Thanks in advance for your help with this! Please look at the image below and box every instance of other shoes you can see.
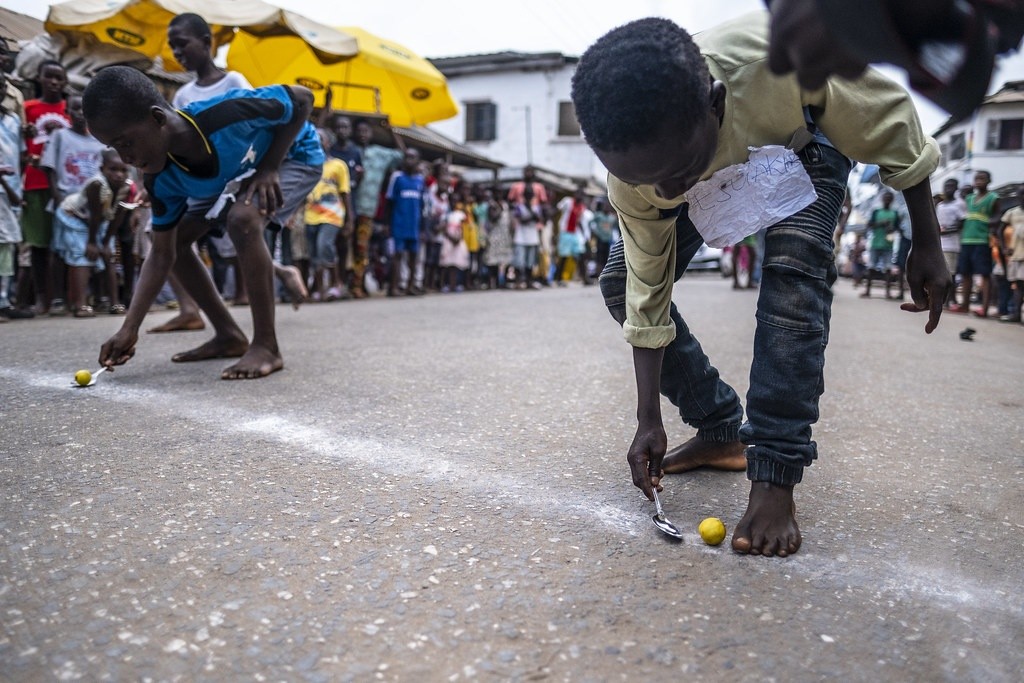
[948,304,969,313]
[971,306,987,318]
[1,305,34,319]
[109,303,126,314]
[73,305,96,316]
[998,313,1021,323]
[47,297,69,316]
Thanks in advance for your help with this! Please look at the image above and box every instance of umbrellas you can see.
[47,3,458,128]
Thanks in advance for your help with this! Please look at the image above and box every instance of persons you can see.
[858,191,900,301]
[57,150,135,317]
[897,201,912,299]
[933,194,940,206]
[146,94,618,311]
[935,179,965,310]
[768,1,1023,96]
[949,169,1002,317]
[143,13,306,334]
[998,190,1023,322]
[849,234,866,288]
[40,95,108,314]
[833,186,851,255]
[958,184,974,200]
[0,37,29,311]
[571,13,954,558]
[732,234,757,291]
[81,65,313,379]
[989,209,1013,316]
[0,72,39,321]
[21,59,73,316]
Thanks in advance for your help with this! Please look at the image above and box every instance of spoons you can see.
[71,365,110,386]
[649,483,684,541]
